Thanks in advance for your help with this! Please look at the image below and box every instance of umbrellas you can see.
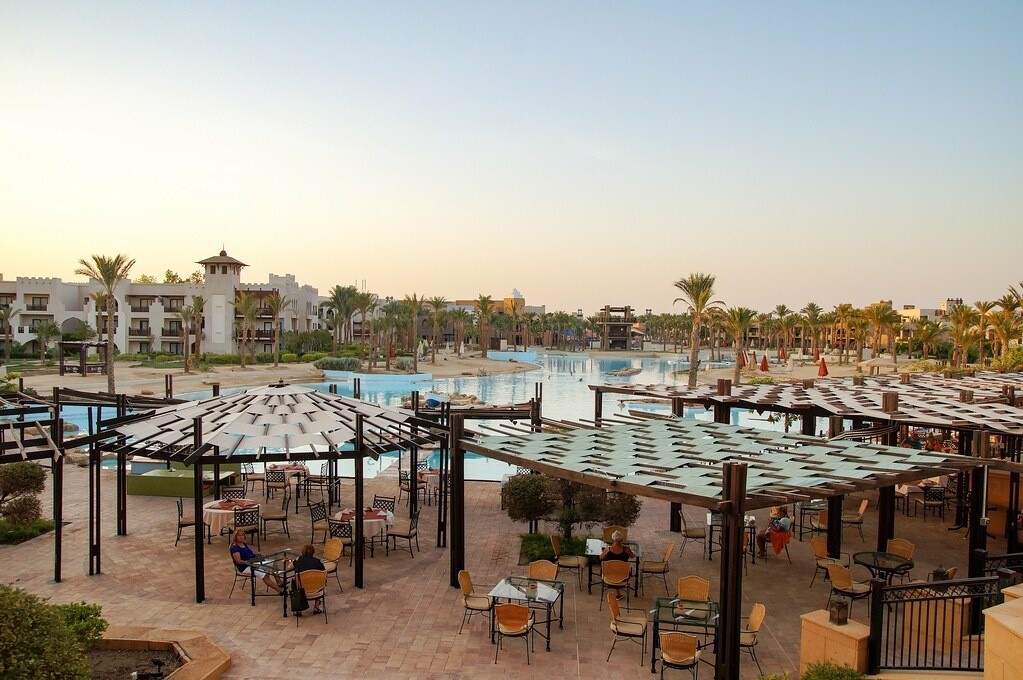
[387,342,396,357]
[759,355,770,374]
[798,348,803,363]
[858,357,900,375]
[817,357,828,379]
[778,348,786,360]
[459,341,464,357]
[446,343,450,351]
[741,350,758,368]
[814,349,819,363]
[417,338,436,360]
[468,339,473,347]
[833,341,845,347]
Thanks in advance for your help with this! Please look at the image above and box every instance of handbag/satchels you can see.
[289,573,309,612]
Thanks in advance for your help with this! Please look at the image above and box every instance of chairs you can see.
[229,538,344,628]
[367,494,396,546]
[550,535,588,591]
[458,570,496,638]
[221,485,245,535]
[387,506,422,558]
[308,499,337,546]
[526,560,558,617]
[397,459,450,507]
[265,468,292,503]
[327,517,366,567]
[307,461,329,497]
[494,604,535,666]
[288,460,306,490]
[599,429,1023,680]
[243,463,267,496]
[174,497,210,546]
[260,491,292,542]
[228,505,261,552]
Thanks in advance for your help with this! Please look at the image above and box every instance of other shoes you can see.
[278,586,289,596]
[279,581,290,588]
[756,554,765,559]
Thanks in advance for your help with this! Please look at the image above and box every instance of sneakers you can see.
[616,594,624,600]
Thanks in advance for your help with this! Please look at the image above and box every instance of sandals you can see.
[293,611,302,617]
[313,607,322,615]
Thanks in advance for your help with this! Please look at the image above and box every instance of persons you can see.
[600,530,636,601]
[923,432,943,455]
[230,529,291,596]
[288,543,328,617]
[756,505,792,558]
[899,434,922,453]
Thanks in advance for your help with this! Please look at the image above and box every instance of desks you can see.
[244,549,332,617]
[852,551,915,612]
[409,468,450,506]
[585,538,642,597]
[650,597,719,680]
[334,508,395,558]
[793,499,844,543]
[705,512,757,564]
[263,465,310,498]
[486,576,564,652]
[203,498,261,551]
[894,479,945,517]
